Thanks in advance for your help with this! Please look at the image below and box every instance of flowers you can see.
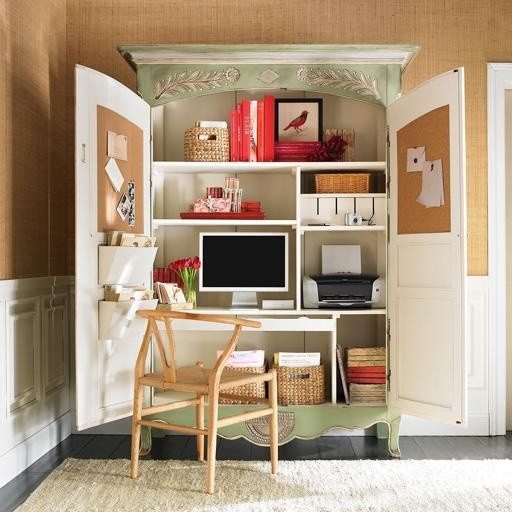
[167,256,200,291]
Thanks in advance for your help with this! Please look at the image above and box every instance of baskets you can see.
[314,173,369,193]
[273,364,324,406]
[184,128,229,162]
[218,360,267,404]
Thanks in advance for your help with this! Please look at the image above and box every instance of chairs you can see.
[129,308,279,494]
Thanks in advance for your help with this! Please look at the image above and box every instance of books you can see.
[243,100,249,161]
[106,232,157,249]
[104,287,155,302]
[230,109,237,162]
[278,351,322,368]
[256,98,264,162]
[215,349,265,368]
[195,120,227,140]
[154,282,186,305]
[346,347,387,407]
[236,102,243,161]
[249,99,257,164]
[263,95,275,162]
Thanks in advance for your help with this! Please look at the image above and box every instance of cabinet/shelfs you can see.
[74,42,469,458]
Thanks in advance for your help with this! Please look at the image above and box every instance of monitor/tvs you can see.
[198,232,289,310]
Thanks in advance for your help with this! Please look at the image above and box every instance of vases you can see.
[181,277,197,308]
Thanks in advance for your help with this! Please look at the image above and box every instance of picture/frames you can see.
[274,97,324,141]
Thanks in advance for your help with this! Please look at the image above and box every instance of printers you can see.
[301,273,385,308]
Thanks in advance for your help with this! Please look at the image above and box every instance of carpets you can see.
[13,457,511,512]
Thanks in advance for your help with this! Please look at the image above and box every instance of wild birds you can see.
[282,110,310,134]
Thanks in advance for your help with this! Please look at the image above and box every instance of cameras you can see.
[344,212,362,227]
